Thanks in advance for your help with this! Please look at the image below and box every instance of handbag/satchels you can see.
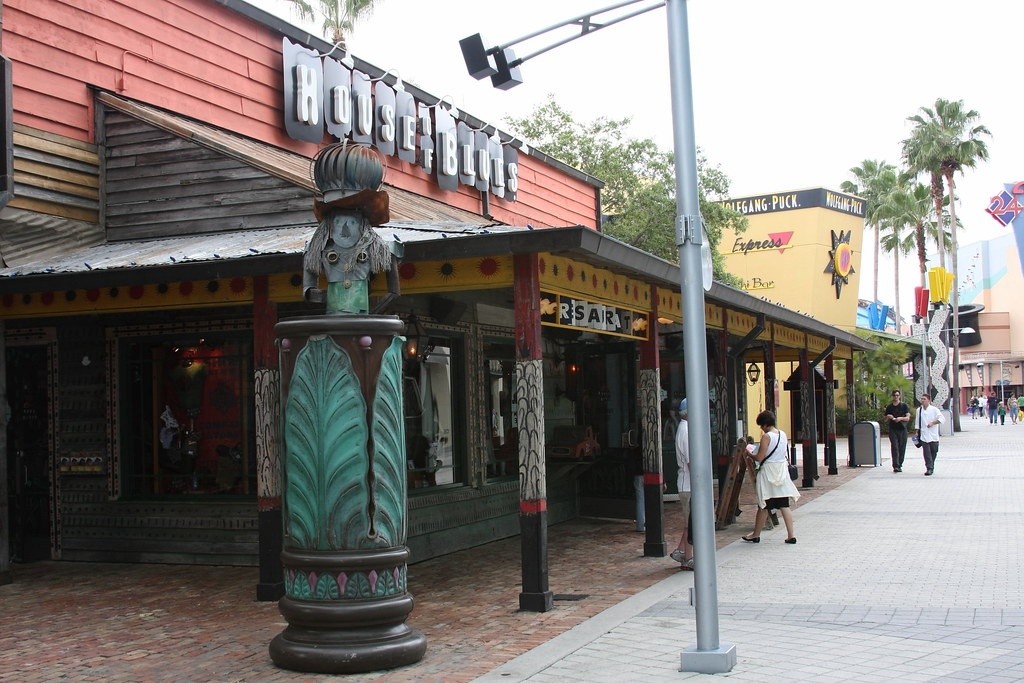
[1017,410,1023,421]
[787,464,798,480]
[915,435,922,448]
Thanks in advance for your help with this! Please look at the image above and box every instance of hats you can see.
[680,398,687,410]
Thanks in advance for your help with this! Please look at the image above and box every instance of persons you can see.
[670,396,700,571]
[884,389,911,472]
[969,392,1024,425]
[914,393,945,476]
[740,410,801,544]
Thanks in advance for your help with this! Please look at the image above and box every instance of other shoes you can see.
[898,467,902,472]
[1001,423,1004,425]
[925,469,933,475]
[894,468,899,472]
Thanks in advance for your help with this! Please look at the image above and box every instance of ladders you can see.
[716,437,779,530]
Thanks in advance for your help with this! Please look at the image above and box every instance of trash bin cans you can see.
[852,420,882,467]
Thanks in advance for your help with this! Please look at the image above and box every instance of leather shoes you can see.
[742,536,760,542]
[785,537,796,544]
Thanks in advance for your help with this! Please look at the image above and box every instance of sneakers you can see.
[681,558,695,570]
[670,549,686,562]
